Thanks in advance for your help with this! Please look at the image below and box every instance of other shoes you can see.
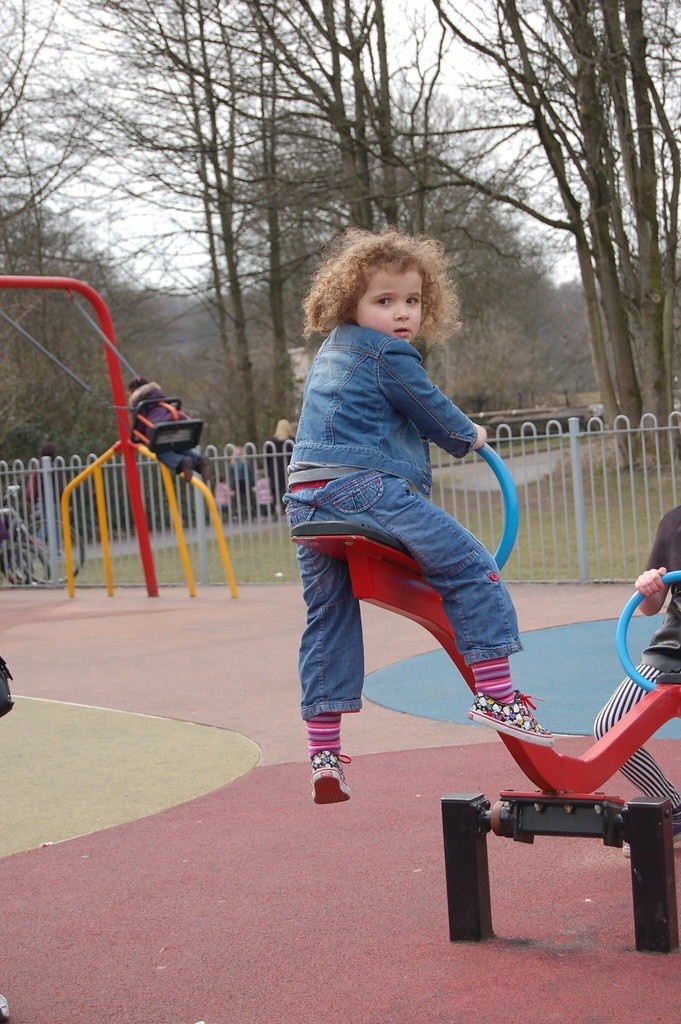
[255,515,264,523]
[266,516,274,524]
[622,805,681,858]
[180,456,193,482]
[197,456,211,481]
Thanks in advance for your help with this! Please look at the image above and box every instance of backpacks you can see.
[0,655,15,719]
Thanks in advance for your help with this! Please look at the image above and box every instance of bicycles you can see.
[0,484,85,586]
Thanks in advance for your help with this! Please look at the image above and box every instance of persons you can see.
[25,444,69,560]
[125,377,213,482]
[0,495,38,584]
[279,226,553,804]
[593,431,681,857]
[211,419,296,523]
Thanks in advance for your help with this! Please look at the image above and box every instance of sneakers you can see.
[310,750,352,804]
[467,689,554,747]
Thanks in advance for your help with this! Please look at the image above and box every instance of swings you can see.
[0,290,204,455]
